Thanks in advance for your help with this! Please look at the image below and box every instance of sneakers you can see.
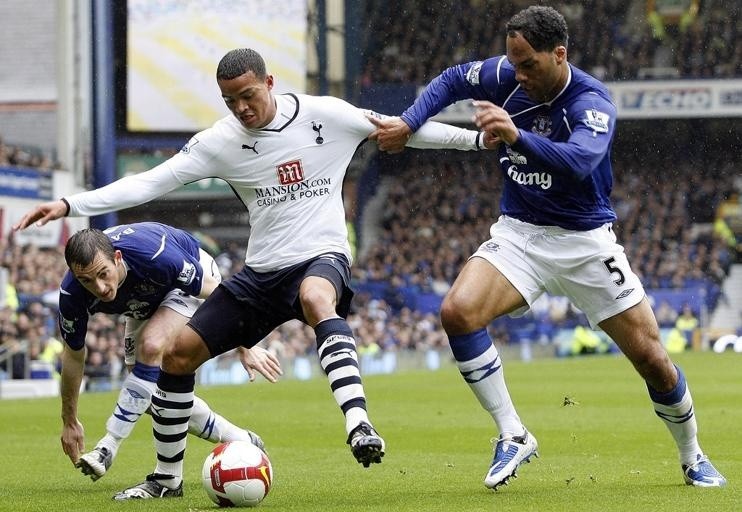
[112,473,183,500]
[248,431,265,454]
[77,447,111,482]
[347,422,385,468]
[485,425,539,491]
[682,454,727,487]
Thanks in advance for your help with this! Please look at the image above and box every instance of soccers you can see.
[203,441,273,506]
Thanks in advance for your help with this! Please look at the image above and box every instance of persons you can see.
[366,2,731,490]
[10,47,505,503]
[58,221,286,483]
